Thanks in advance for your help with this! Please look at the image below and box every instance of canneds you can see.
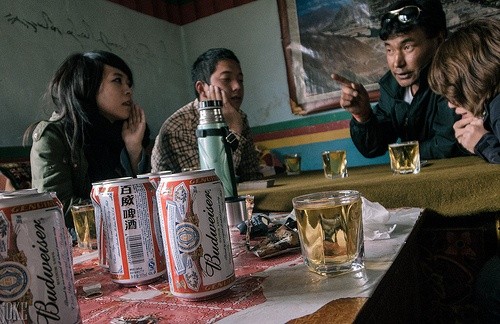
[90,168,235,302]
[0,187,80,324]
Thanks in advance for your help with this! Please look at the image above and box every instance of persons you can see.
[23,49,148,229]
[150,46,269,182]
[426,17,500,164]
[331,1,462,160]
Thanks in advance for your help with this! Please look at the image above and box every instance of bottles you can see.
[196,101,238,202]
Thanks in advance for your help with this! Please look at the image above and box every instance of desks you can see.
[0,156,500,324]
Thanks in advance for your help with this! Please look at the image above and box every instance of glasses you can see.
[381,6,426,25]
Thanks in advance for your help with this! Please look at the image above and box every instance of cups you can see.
[388,141,421,175]
[225,197,247,226]
[292,190,365,277]
[282,152,302,175]
[70,202,97,250]
[321,149,348,179]
